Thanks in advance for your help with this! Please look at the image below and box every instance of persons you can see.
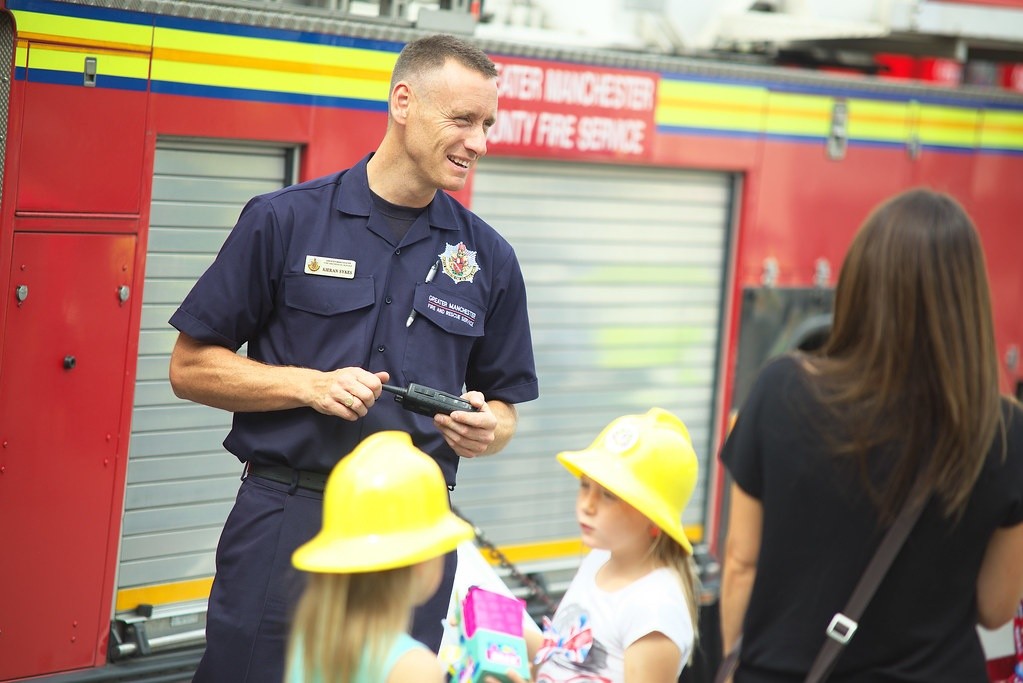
[281,431,474,683]
[481,406,703,683]
[717,188,1023,683]
[168,36,540,683]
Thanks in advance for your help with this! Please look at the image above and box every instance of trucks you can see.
[1,1,1023,683]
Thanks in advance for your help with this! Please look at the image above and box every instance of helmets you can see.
[557,407,698,556]
[291,428,475,575]
